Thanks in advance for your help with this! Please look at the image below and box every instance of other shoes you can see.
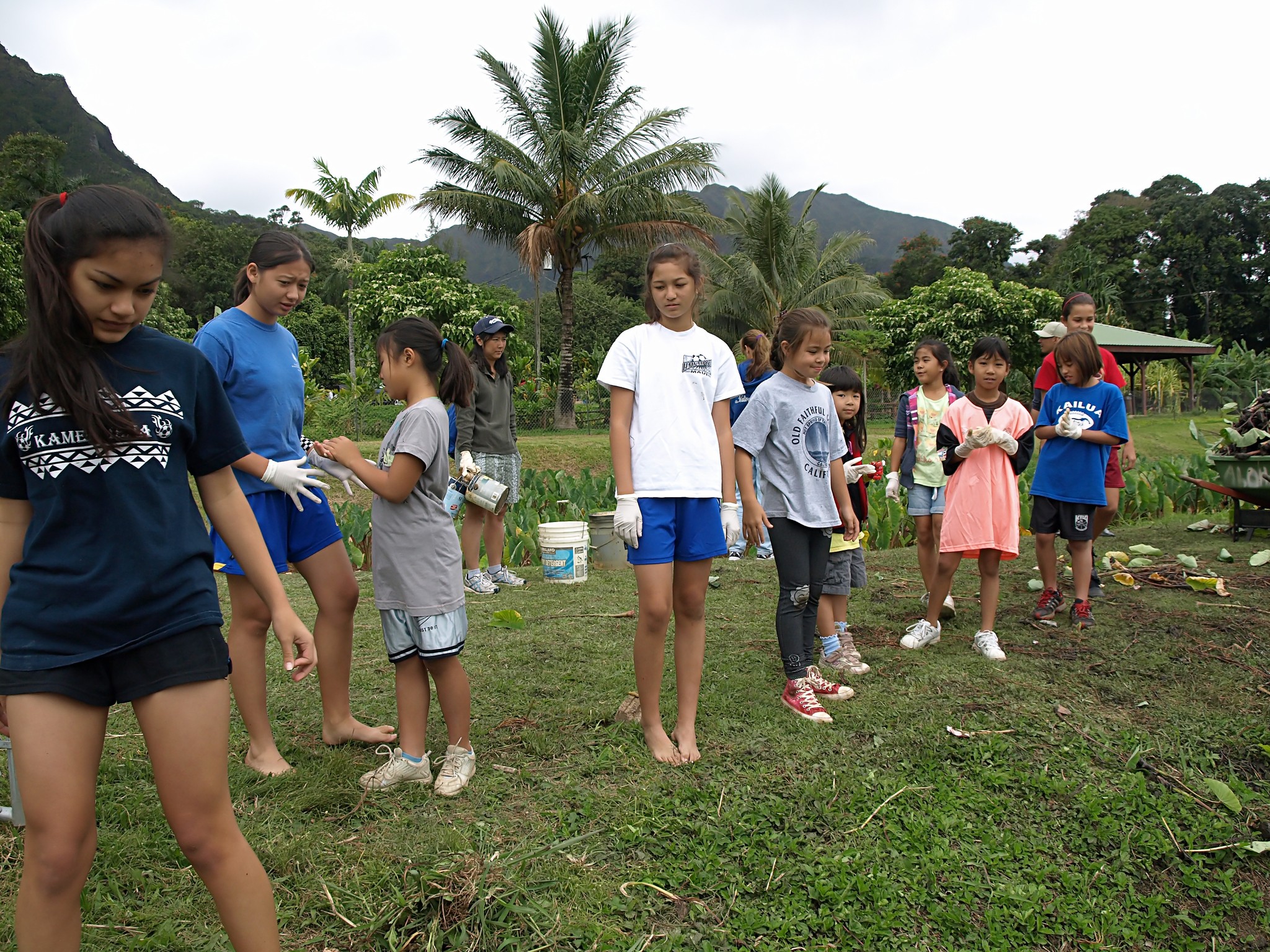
[1098,529,1115,537]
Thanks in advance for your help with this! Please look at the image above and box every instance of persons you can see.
[731,307,860,723]
[454,313,527,594]
[885,339,965,620]
[899,336,1034,661]
[1029,321,1115,537]
[729,329,779,560]
[312,317,477,797]
[596,242,740,767]
[818,365,883,675]
[1028,331,1129,628]
[0,183,319,952]
[1032,291,1136,598]
[191,231,397,777]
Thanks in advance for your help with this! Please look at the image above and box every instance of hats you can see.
[473,316,514,339]
[1032,322,1067,338]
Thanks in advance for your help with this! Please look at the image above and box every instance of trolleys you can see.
[1180,453,1270,540]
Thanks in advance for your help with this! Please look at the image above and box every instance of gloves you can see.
[867,461,883,480]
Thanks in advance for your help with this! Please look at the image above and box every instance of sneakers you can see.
[358,745,432,790]
[972,629,1007,661]
[899,619,941,649]
[1031,586,1068,621]
[1070,597,1096,629]
[1066,543,1097,569]
[1088,570,1105,597]
[805,665,854,700]
[818,647,871,675]
[489,566,526,586]
[728,551,744,560]
[756,554,774,560]
[433,736,476,796]
[837,632,861,660]
[463,570,501,595]
[780,677,834,724]
[920,590,957,618]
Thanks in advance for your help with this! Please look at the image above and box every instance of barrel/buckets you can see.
[443,475,469,519]
[465,472,510,515]
[588,511,632,571]
[537,499,590,584]
